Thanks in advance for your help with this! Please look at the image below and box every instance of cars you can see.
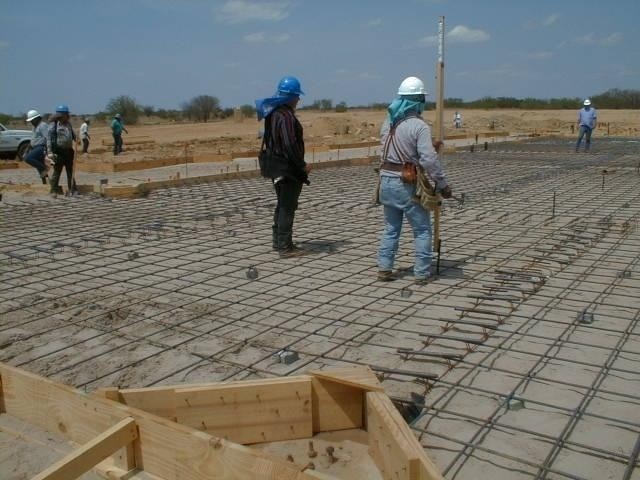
[0,121,36,162]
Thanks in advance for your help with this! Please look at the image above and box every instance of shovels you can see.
[65,127,80,198]
[57,185,64,195]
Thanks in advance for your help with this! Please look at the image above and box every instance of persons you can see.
[252,74,313,261]
[373,74,452,286]
[453,110,463,129]
[45,103,82,196]
[78,118,91,153]
[109,112,130,157]
[20,108,50,186]
[574,98,596,153]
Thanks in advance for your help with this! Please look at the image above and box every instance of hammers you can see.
[450,193,465,209]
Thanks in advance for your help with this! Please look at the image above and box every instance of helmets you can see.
[397,76,429,95]
[84,118,90,122]
[26,110,40,121]
[584,99,591,105]
[56,104,71,113]
[277,76,305,95]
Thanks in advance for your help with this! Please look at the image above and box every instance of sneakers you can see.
[377,270,393,281]
[40,165,50,178]
[415,277,428,286]
[50,190,82,199]
[272,244,305,259]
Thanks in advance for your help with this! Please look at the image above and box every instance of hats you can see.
[112,114,123,120]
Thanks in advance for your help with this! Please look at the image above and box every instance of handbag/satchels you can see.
[401,163,442,211]
[258,147,297,179]
[372,178,381,203]
